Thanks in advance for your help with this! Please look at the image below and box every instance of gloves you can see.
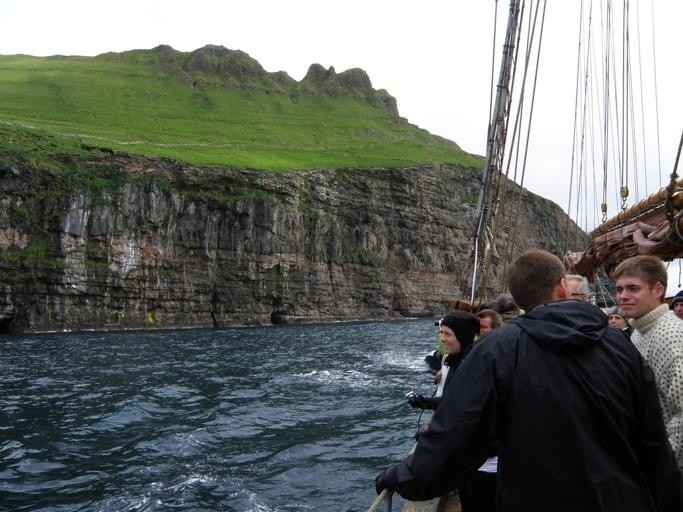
[372,464,397,499]
[407,394,424,410]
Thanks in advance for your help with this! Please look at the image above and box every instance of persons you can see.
[374,249,682,511]
[399,271,682,416]
[616,252,682,461]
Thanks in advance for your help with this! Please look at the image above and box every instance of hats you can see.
[606,305,628,325]
[440,309,480,346]
[671,289,683,309]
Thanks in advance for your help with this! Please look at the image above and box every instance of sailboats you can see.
[364,0,682,510]
